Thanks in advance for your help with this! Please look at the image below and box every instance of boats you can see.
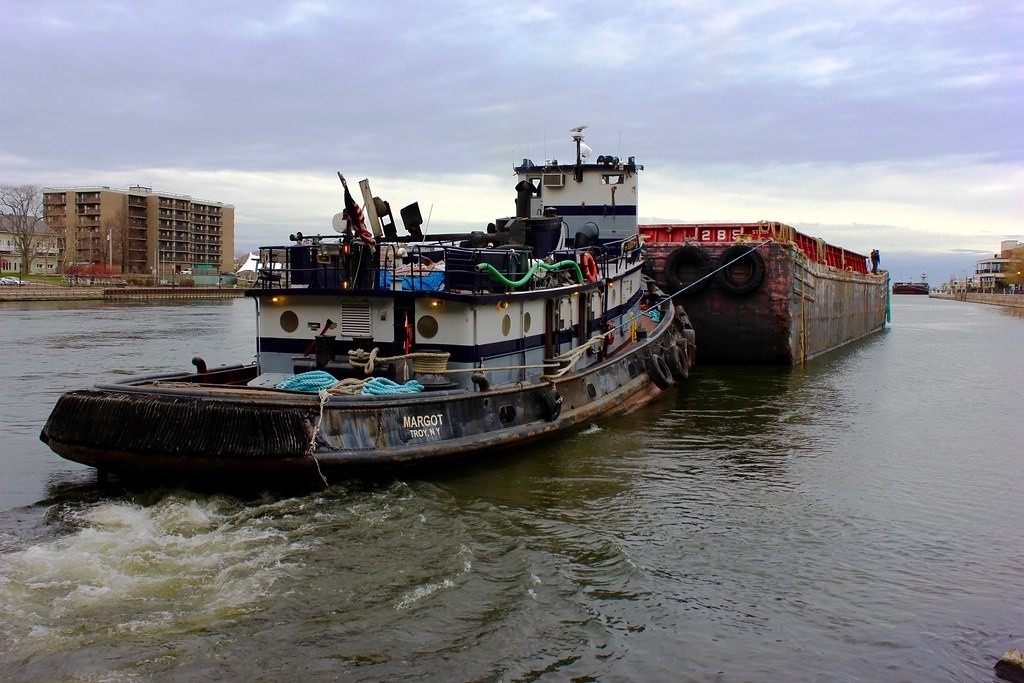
[39,128,697,480]
[892,282,929,295]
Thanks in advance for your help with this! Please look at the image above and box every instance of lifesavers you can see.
[582,252,599,284]
[605,322,616,344]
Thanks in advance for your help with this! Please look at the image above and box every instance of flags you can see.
[339,187,376,246]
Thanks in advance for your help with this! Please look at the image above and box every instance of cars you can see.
[1,276,26,286]
[181,268,192,275]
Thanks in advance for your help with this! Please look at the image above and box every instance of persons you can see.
[871,249,875,273]
[873,250,880,275]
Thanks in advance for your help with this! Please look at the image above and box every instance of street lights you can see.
[1018,272,1020,290]
